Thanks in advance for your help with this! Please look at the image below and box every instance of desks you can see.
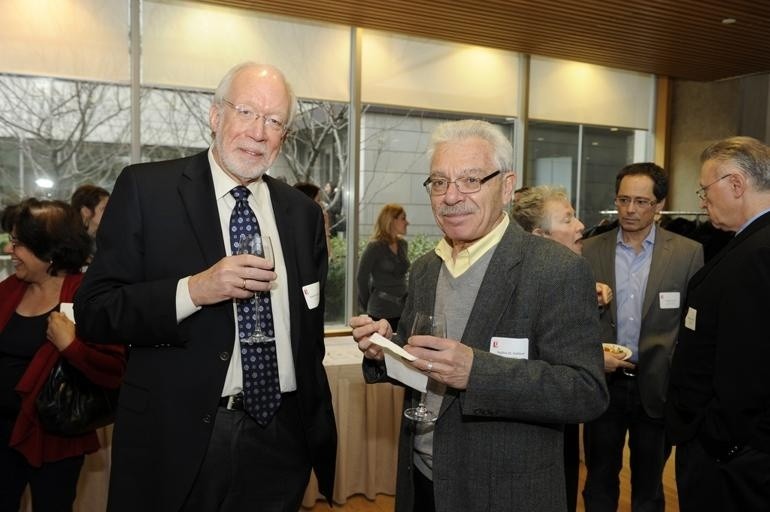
[304,335,406,506]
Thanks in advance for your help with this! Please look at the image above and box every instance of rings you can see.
[427,359,433,373]
[243,278,247,288]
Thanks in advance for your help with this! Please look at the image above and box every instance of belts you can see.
[218,390,297,412]
[616,363,639,377]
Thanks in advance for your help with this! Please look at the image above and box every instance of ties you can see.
[229,185,282,429]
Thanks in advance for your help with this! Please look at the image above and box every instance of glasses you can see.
[695,172,733,201]
[612,194,657,208]
[222,97,285,131]
[8,233,25,249]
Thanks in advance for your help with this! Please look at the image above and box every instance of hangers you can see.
[590,208,718,237]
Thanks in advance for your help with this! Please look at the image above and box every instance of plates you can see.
[603,341,632,360]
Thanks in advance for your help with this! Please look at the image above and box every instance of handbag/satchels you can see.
[33,359,120,438]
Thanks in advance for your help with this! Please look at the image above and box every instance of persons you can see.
[355,206,412,333]
[582,164,704,511]
[70,185,111,272]
[0,199,126,512]
[290,182,331,260]
[511,183,635,373]
[672,135,770,512]
[349,118,609,512]
[72,62,338,512]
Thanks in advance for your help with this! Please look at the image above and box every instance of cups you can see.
[405,313,447,424]
[239,235,276,344]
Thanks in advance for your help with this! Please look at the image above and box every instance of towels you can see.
[423,169,501,196]
